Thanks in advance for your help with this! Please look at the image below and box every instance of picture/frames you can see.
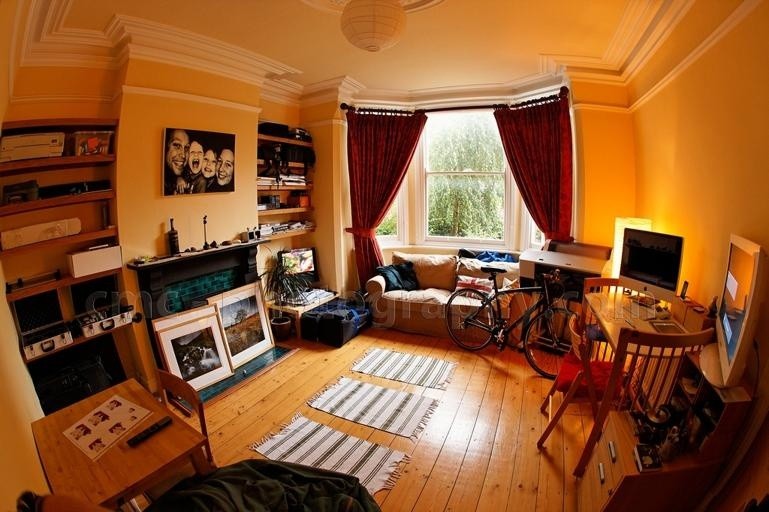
[157,313,235,393]
[151,304,220,333]
[207,281,275,368]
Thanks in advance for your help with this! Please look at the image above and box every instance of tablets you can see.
[650,321,684,335]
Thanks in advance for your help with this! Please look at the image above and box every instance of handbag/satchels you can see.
[300,296,373,348]
[375,261,420,293]
[258,139,316,181]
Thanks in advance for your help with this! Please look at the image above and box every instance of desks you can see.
[573,278,717,479]
[32,379,208,509]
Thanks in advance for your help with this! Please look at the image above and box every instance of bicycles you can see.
[444,266,579,379]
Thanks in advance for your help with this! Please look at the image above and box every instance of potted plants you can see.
[265,264,310,340]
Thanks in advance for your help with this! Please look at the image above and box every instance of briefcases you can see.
[12,288,74,361]
[69,274,135,339]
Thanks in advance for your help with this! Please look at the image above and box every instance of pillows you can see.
[456,276,495,299]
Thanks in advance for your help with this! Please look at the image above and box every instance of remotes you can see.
[126,416,173,446]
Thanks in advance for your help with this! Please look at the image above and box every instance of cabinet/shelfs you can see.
[0,115,135,377]
[519,249,604,353]
[260,121,317,268]
[577,353,752,512]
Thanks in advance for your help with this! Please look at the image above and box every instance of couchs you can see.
[364,251,522,341]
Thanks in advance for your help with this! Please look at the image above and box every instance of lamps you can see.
[340,0,407,52]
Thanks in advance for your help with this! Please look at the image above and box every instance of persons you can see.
[165,129,235,193]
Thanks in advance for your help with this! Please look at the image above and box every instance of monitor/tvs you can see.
[699,233,767,387]
[617,226,684,300]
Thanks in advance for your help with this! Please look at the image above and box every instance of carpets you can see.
[308,376,441,439]
[351,347,459,391]
[247,413,409,497]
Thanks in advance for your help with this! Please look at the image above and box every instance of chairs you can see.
[537,313,636,448]
[130,367,217,512]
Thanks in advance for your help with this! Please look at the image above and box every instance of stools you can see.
[268,290,338,340]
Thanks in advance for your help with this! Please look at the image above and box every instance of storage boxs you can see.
[70,131,112,156]
[63,246,123,279]
